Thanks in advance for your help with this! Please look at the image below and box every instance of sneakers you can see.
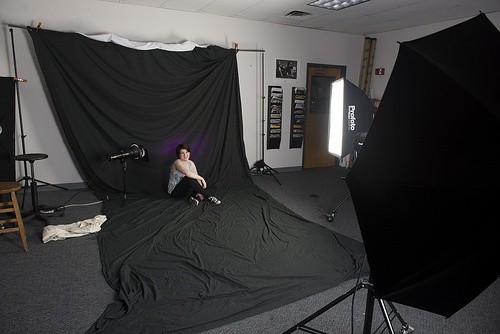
[189,197,199,206]
[208,196,221,205]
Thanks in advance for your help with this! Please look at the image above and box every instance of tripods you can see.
[9,28,68,209]
[249,53,282,186]
[105,159,140,206]
[282,271,415,334]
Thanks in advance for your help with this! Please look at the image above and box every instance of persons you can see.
[168,143,221,206]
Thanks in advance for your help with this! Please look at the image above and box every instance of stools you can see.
[0,182,31,253]
[14,153,65,227]
